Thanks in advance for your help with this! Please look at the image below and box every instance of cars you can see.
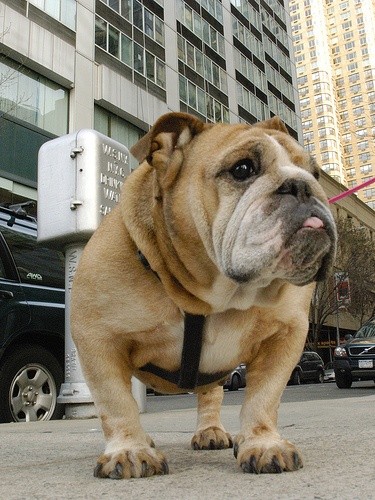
[225,364,249,391]
[284,352,325,386]
[324,369,336,381]
[331,322,375,388]
[0,206,65,423]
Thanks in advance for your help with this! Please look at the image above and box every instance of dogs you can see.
[68,111,340,481]
